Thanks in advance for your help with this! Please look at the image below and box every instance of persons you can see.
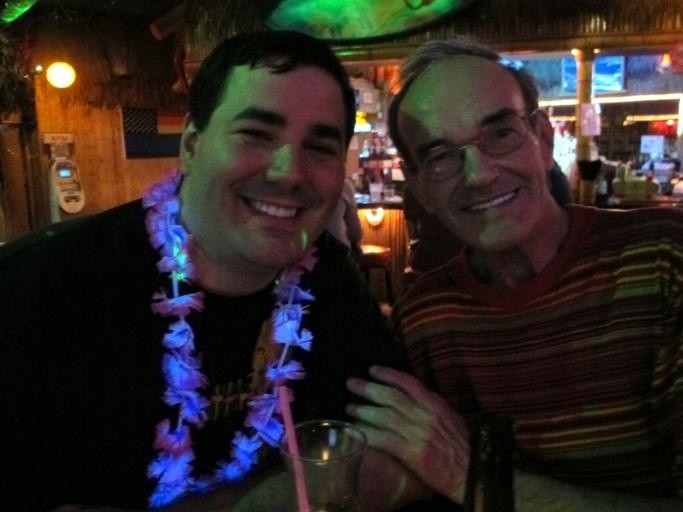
[341,35,683,512]
[397,183,460,303]
[546,156,573,211]
[1,29,439,512]
[325,179,370,284]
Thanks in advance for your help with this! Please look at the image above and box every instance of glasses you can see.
[403,106,540,184]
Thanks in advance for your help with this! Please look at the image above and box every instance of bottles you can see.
[359,138,392,166]
[461,413,517,512]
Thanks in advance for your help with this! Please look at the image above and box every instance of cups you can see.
[278,421,367,511]
[369,183,395,202]
[579,179,596,205]
